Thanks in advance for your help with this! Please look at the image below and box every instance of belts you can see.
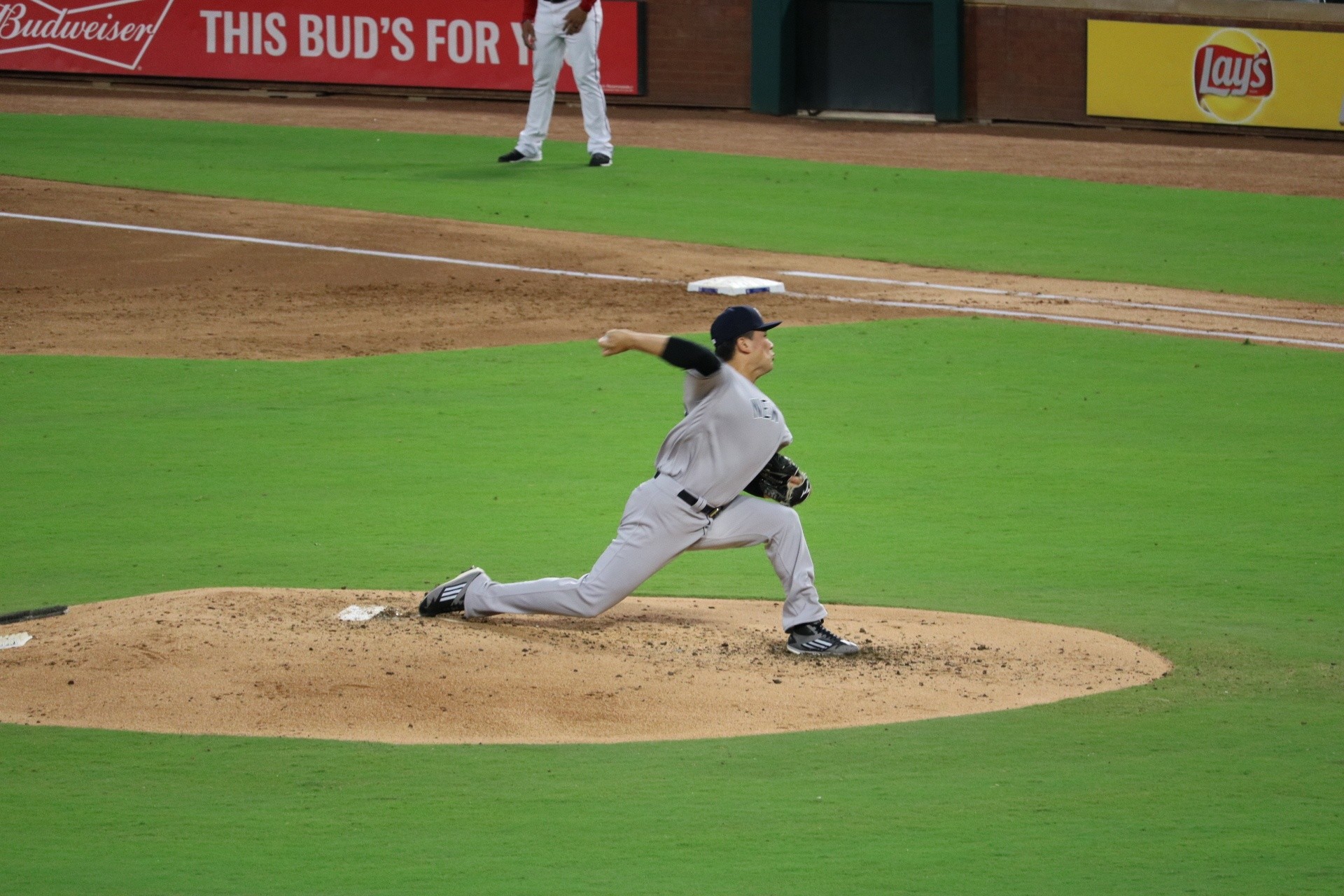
[655,470,724,520]
[544,0,568,3]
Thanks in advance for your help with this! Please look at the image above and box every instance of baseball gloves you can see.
[751,451,811,508]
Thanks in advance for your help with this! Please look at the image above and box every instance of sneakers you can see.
[786,618,859,656]
[419,567,486,616]
[498,149,542,163]
[588,153,612,167]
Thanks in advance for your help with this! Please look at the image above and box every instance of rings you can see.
[575,25,578,28]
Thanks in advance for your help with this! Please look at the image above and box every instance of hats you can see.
[711,306,782,347]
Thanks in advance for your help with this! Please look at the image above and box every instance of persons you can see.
[419,306,858,655]
[498,0,613,166]
